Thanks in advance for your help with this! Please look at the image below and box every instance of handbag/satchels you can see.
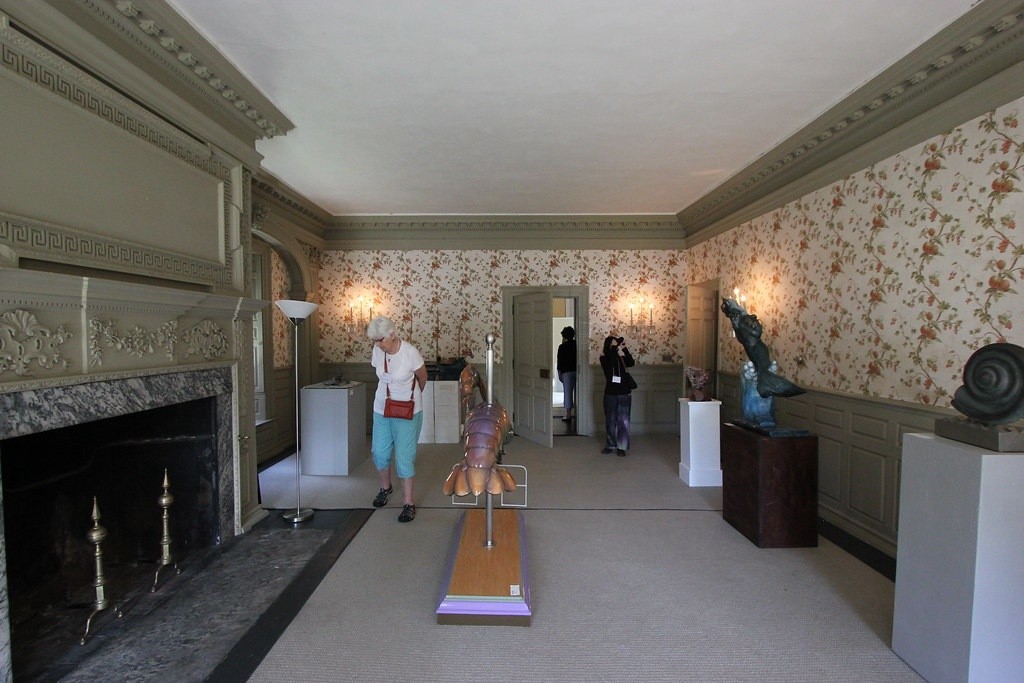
[383,397,415,421]
[620,356,638,389]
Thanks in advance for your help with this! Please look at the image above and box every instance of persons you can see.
[599,335,637,457]
[367,316,429,522]
[557,326,576,422]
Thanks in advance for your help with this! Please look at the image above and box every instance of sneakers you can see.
[373,483,393,507]
[398,502,416,522]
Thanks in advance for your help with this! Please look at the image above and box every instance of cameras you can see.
[614,337,624,346]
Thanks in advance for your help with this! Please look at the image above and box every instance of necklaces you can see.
[387,337,399,363]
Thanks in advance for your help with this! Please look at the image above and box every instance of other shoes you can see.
[600,447,614,454]
[617,449,626,457]
[561,415,571,421]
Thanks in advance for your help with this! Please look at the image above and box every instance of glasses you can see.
[372,336,385,343]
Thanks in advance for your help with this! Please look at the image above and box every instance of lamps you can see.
[273,299,319,524]
[346,297,374,333]
[627,296,656,335]
[728,287,762,338]
